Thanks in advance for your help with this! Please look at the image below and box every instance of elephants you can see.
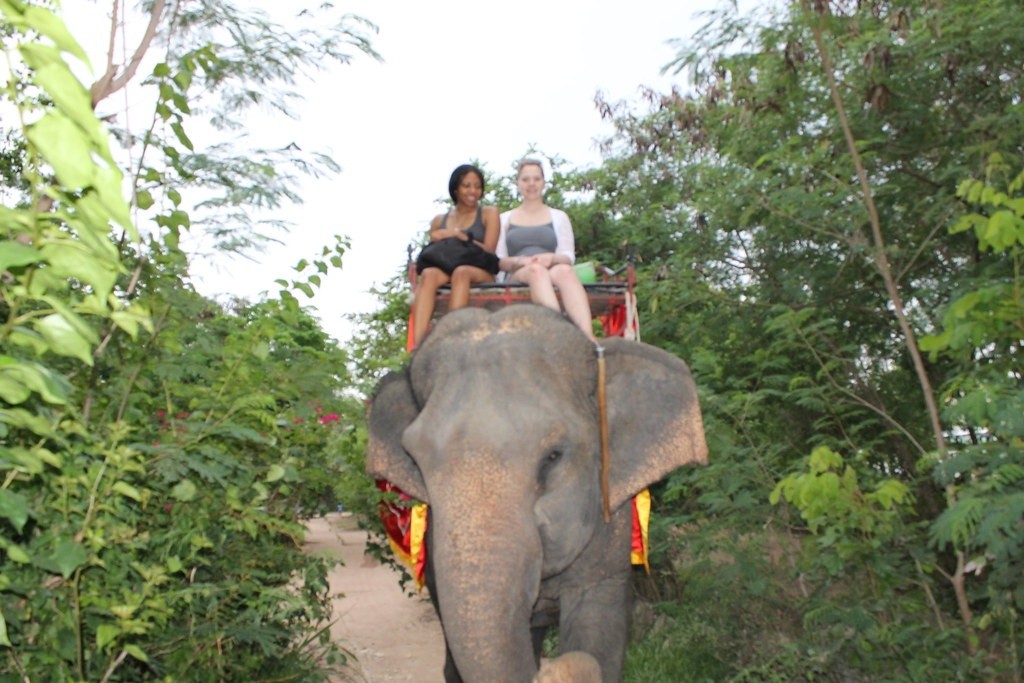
[362,300,711,683]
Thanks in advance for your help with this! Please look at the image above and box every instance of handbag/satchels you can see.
[415,232,499,274]
[572,261,596,282]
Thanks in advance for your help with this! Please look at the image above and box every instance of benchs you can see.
[407,260,640,350]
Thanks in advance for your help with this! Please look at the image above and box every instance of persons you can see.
[413,165,500,350]
[495,160,600,346]
